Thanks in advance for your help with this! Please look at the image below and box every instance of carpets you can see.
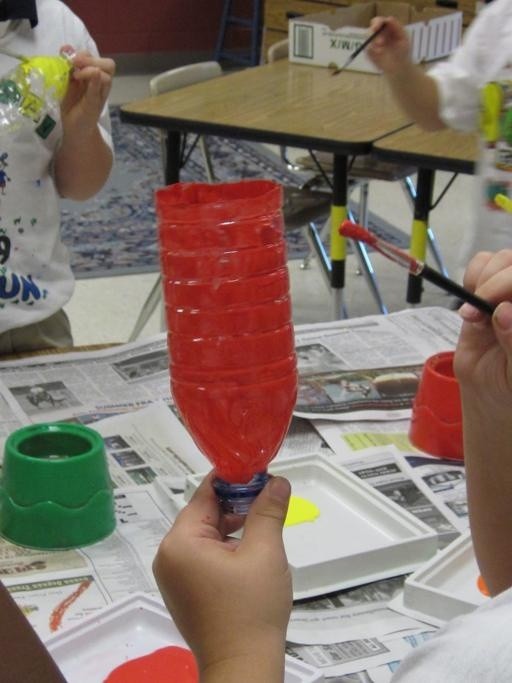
[56,101,412,280]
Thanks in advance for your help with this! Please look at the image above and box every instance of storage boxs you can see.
[286,1,464,74]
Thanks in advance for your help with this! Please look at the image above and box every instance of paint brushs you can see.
[338,219,495,317]
[332,21,388,76]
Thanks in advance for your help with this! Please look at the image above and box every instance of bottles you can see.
[407,351,464,461]
[154,179,299,515]
[0,51,78,143]
[0,422,117,551]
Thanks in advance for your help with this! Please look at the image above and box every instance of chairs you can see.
[125,57,386,347]
[265,35,454,294]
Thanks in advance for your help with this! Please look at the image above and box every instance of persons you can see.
[152,247,512,683]
[0,0,115,354]
[365,0,512,310]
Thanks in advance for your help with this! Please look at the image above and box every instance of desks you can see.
[2,337,512,681]
[116,55,414,320]
[372,118,496,312]
